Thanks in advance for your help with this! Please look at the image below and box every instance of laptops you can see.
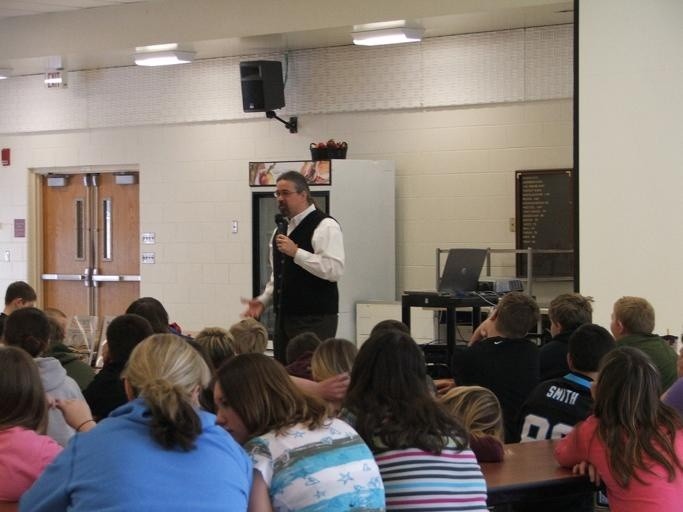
[403,248,488,296]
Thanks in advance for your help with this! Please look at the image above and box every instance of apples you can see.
[258,171,273,186]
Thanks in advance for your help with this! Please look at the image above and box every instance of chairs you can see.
[63,314,97,366]
[95,316,117,374]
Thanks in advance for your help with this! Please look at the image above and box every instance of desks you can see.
[403,295,498,378]
[478,440,575,494]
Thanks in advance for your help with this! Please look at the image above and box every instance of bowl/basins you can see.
[310,140,348,161]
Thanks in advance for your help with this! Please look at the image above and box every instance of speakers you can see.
[239,60,285,112]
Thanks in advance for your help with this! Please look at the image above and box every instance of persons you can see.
[0,282,682,510]
[240,172,345,370]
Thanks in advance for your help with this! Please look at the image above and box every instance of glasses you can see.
[273,189,303,198]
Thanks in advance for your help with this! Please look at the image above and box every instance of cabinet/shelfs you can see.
[356,302,548,352]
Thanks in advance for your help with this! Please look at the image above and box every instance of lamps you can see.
[351,28,425,47]
[135,51,197,67]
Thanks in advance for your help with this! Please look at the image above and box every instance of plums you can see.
[317,139,347,148]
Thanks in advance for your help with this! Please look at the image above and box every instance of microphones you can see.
[274,214,289,263]
